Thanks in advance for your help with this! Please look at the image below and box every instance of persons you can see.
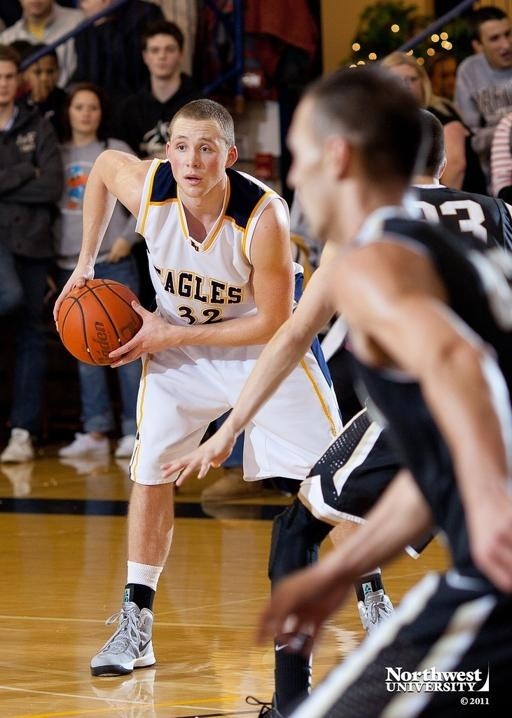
[46,82,154,461]
[156,106,512,716]
[24,41,59,106]
[49,97,395,678]
[376,51,491,197]
[111,20,202,159]
[1,45,66,463]
[246,67,512,716]
[0,1,85,93]
[453,5,512,133]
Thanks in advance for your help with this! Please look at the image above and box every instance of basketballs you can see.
[58,279,141,364]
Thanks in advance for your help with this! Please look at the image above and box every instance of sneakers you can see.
[58,432,109,457]
[90,601,156,677]
[115,435,136,458]
[357,595,395,633]
[0,428,35,462]
[245,693,311,718]
